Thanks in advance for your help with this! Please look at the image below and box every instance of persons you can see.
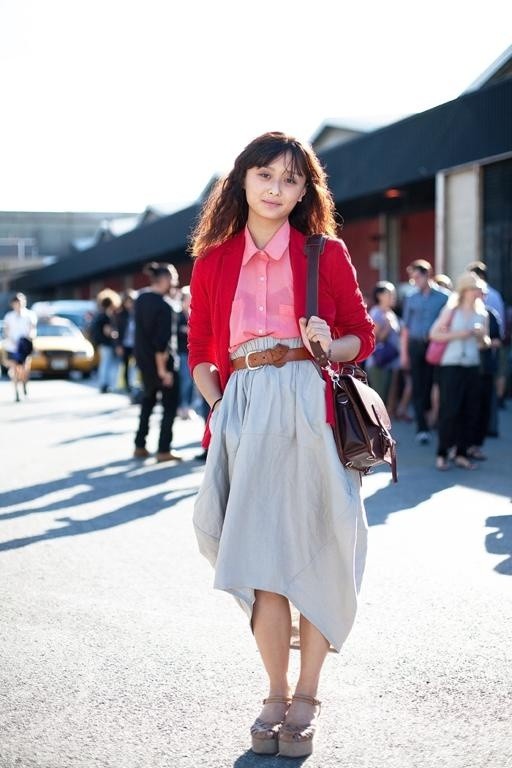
[362,259,510,473]
[0,290,38,405]
[84,263,211,464]
[185,129,376,759]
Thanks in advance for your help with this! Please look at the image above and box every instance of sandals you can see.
[278,694,322,759]
[250,697,291,755]
[436,447,487,471]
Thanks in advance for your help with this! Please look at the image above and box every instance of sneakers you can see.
[415,431,430,444]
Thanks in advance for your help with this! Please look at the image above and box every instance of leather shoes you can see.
[135,447,151,457]
[195,450,207,460]
[157,451,182,462]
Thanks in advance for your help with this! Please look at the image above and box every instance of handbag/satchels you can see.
[425,305,457,366]
[304,234,398,484]
[372,339,398,366]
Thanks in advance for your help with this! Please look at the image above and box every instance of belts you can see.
[232,344,312,372]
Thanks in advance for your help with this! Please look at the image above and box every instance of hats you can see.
[456,271,486,293]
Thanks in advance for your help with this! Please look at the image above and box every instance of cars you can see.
[34,300,112,369]
[0,316,97,379]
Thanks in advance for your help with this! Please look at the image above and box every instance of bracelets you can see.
[327,348,332,360]
[212,397,222,412]
